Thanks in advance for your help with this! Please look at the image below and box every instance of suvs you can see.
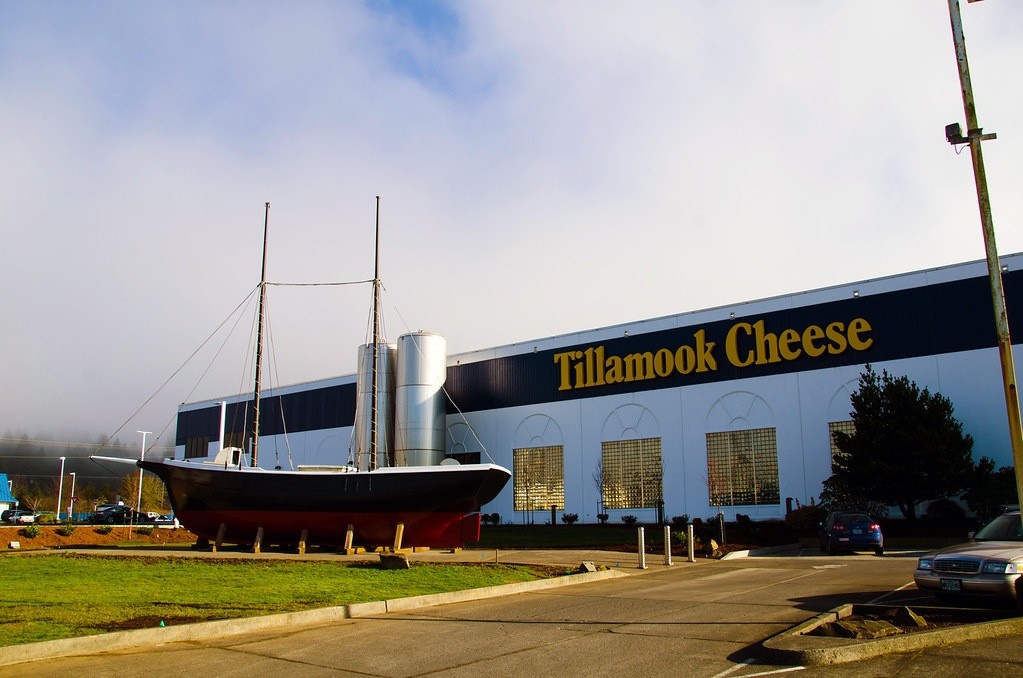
[8,511,35,525]
[1,510,24,522]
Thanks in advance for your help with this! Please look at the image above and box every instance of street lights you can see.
[69,472,75,522]
[8,480,13,492]
[57,456,66,520]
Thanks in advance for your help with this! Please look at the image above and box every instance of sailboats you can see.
[87,195,512,549]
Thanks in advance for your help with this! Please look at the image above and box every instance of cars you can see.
[88,506,148,524]
[914,510,1023,616]
[819,511,885,556]
